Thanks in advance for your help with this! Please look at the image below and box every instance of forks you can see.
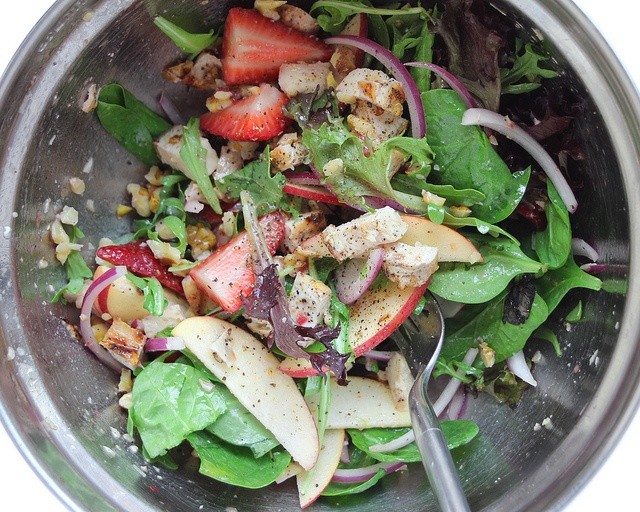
[389,291,470,512]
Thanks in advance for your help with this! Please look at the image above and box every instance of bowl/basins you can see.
[0,0,640,512]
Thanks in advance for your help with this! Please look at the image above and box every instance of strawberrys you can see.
[200,88,288,141]
[190,213,281,316]
[224,9,330,84]
